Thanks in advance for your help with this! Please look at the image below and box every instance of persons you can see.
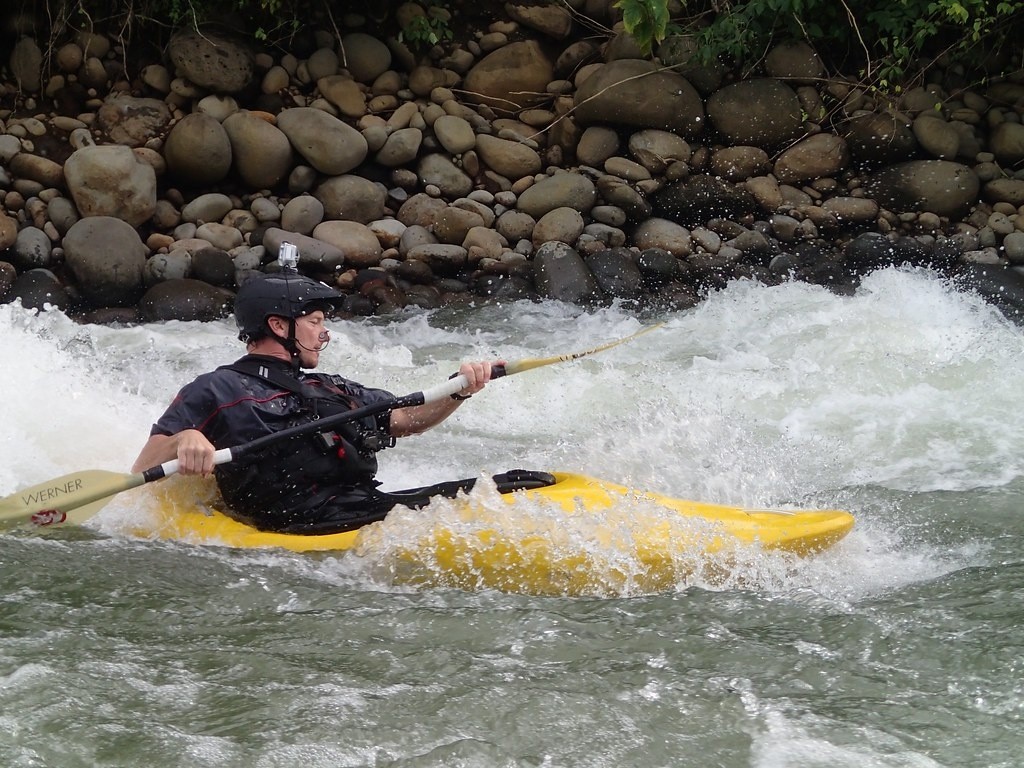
[129,262,510,532]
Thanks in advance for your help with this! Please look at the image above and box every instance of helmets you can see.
[234,269,344,336]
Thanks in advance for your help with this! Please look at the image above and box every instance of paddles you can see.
[0,321,665,538]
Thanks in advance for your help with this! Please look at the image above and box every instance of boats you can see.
[6,469,857,600]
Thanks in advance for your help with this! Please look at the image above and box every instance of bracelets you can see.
[447,371,473,401]
